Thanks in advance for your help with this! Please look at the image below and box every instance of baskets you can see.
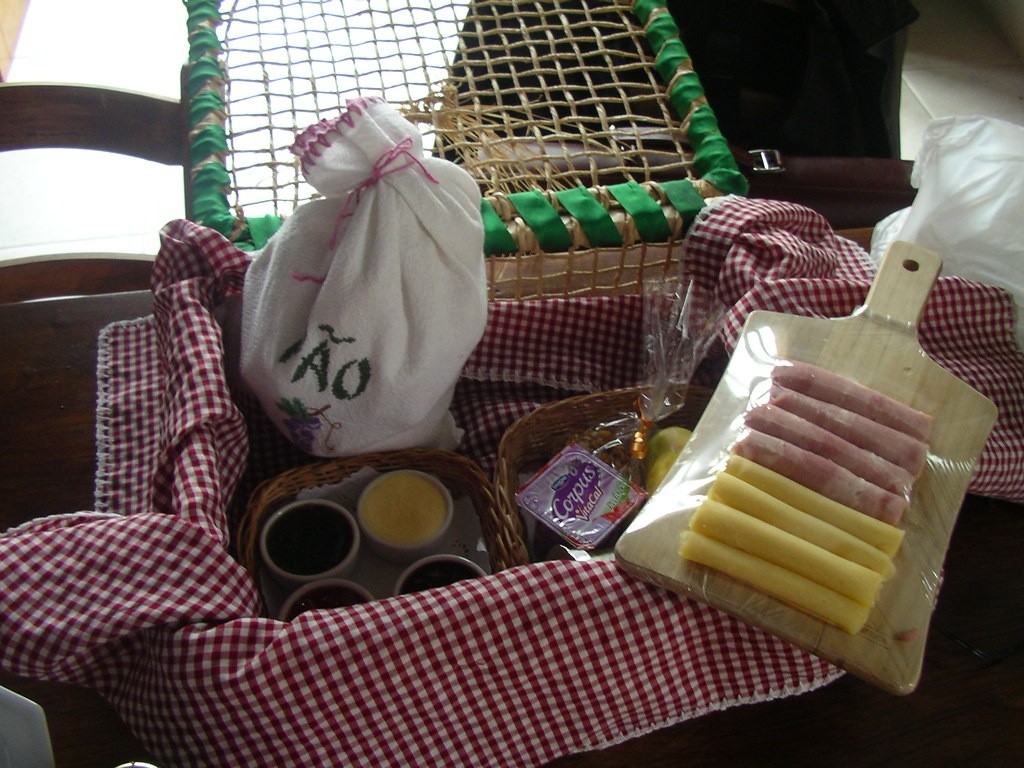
[238,446,527,610]
[493,382,716,565]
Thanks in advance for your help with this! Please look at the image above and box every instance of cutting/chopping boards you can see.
[613,242,998,697]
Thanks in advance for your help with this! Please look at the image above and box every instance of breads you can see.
[570,428,644,487]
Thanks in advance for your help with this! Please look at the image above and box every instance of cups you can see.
[258,499,362,582]
[279,579,377,628]
[357,469,449,559]
[394,552,487,598]
